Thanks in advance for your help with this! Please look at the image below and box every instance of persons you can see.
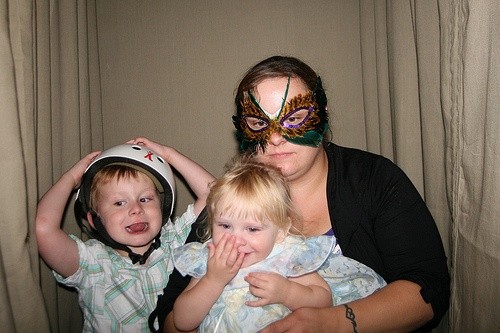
[148,56,451,333]
[173,158,332,333]
[35,137,219,333]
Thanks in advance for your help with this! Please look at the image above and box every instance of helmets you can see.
[75,143,176,228]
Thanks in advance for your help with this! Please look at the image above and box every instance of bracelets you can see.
[343,304,358,333]
